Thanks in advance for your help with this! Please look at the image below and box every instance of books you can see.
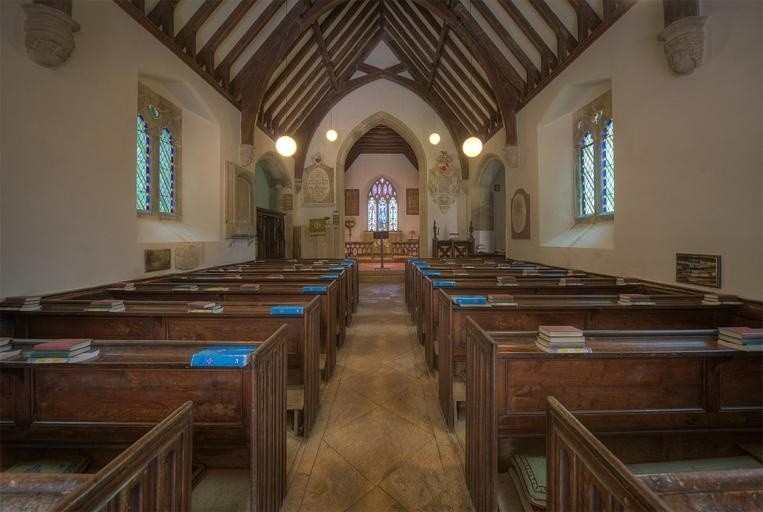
[538,324,583,336]
[188,344,256,368]
[533,340,593,355]
[0,337,100,365]
[406,250,743,314]
[0,253,359,316]
[535,336,587,348]
[538,333,586,343]
[717,325,762,339]
[717,333,762,346]
[717,338,762,352]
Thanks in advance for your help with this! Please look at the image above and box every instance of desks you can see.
[436,290,745,432]
[3,296,323,441]
[39,258,359,383]
[0,400,192,512]
[404,257,718,376]
[544,396,760,510]
[0,321,292,512]
[464,317,763,511]
